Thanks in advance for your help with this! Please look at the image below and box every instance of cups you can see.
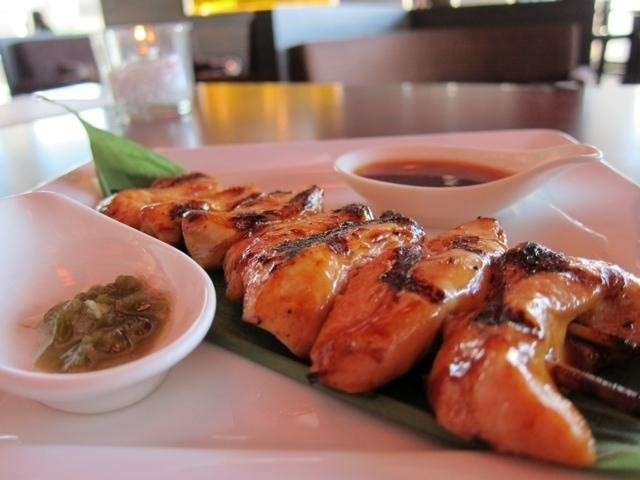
[86,20,196,129]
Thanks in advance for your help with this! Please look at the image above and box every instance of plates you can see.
[0,129,640,480]
[0,190,217,415]
[333,143,603,230]
